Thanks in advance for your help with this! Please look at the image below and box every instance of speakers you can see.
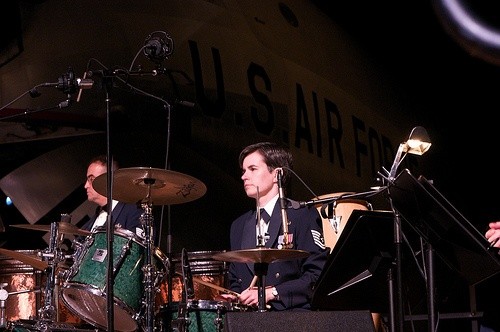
[221,310,378,332]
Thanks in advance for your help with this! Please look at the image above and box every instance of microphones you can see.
[277,170,289,233]
[182,249,195,299]
[146,39,168,56]
[45,78,94,89]
[0,289,8,327]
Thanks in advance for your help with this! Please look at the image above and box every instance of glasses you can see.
[87,175,95,185]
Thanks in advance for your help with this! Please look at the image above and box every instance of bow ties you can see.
[255,208,270,225]
[95,204,108,217]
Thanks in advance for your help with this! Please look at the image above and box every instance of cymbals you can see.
[0,249,70,274]
[210,248,313,264]
[8,223,94,236]
[91,167,207,206]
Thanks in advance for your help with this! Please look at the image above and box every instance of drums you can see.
[57,225,172,332]
[150,250,226,313]
[0,249,82,324]
[305,189,374,256]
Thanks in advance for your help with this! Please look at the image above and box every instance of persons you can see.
[65,154,155,255]
[485,221,500,248]
[220,142,332,311]
